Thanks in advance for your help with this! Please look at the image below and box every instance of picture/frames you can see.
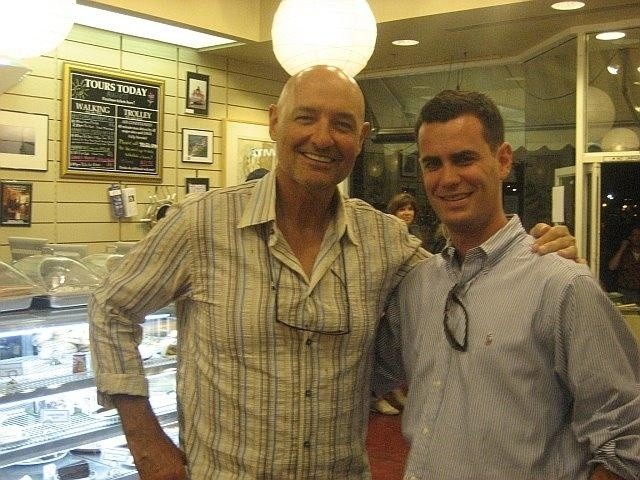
[62,62,166,185]
[181,69,214,194]
[221,117,278,188]
[0,108,49,227]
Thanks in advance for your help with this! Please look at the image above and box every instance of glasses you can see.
[274,261,351,336]
[442,282,469,352]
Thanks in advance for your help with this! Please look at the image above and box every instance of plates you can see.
[54,467,98,480]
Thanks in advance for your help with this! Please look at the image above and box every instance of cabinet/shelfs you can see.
[0,308,179,479]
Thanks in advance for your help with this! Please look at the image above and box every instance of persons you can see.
[364,86,640,480]
[367,192,425,417]
[607,223,639,305]
[83,62,593,480]
